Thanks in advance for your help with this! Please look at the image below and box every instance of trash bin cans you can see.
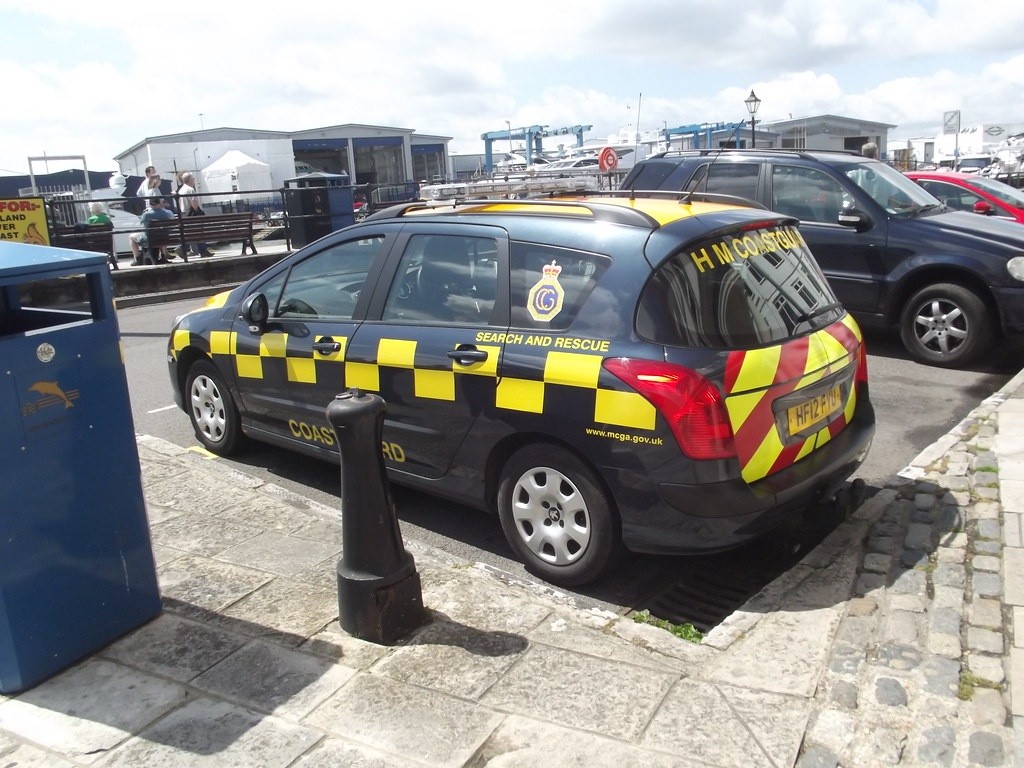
[0,240,164,696]
[283,171,357,249]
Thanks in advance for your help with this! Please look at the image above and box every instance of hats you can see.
[149,199,159,205]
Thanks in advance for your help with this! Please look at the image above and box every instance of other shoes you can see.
[190,247,199,254]
[130,256,142,266]
[174,245,183,258]
[201,249,216,258]
[155,256,172,264]
[162,253,176,259]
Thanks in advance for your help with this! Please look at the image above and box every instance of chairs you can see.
[417,236,473,320]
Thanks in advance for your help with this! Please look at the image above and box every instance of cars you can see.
[902,171,1024,227]
[265,210,289,227]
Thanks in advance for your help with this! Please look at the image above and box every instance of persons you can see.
[174,170,215,260]
[46,206,67,228]
[846,142,899,208]
[86,201,113,223]
[129,166,176,266]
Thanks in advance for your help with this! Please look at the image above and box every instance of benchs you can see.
[141,211,258,264]
[48,222,119,270]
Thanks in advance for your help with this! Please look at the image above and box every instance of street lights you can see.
[505,119,515,170]
[745,88,761,148]
[193,148,203,204]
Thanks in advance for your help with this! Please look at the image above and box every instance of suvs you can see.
[615,146,1024,368]
[165,189,875,587]
